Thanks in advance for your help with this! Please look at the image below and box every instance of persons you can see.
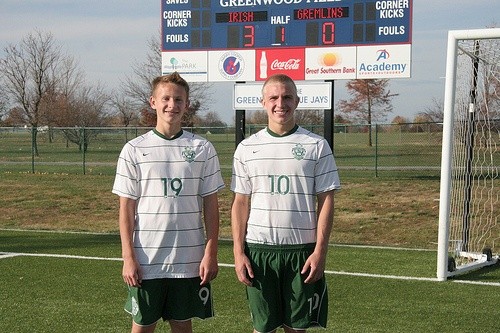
[231,75,340,333]
[110,72,225,332]
